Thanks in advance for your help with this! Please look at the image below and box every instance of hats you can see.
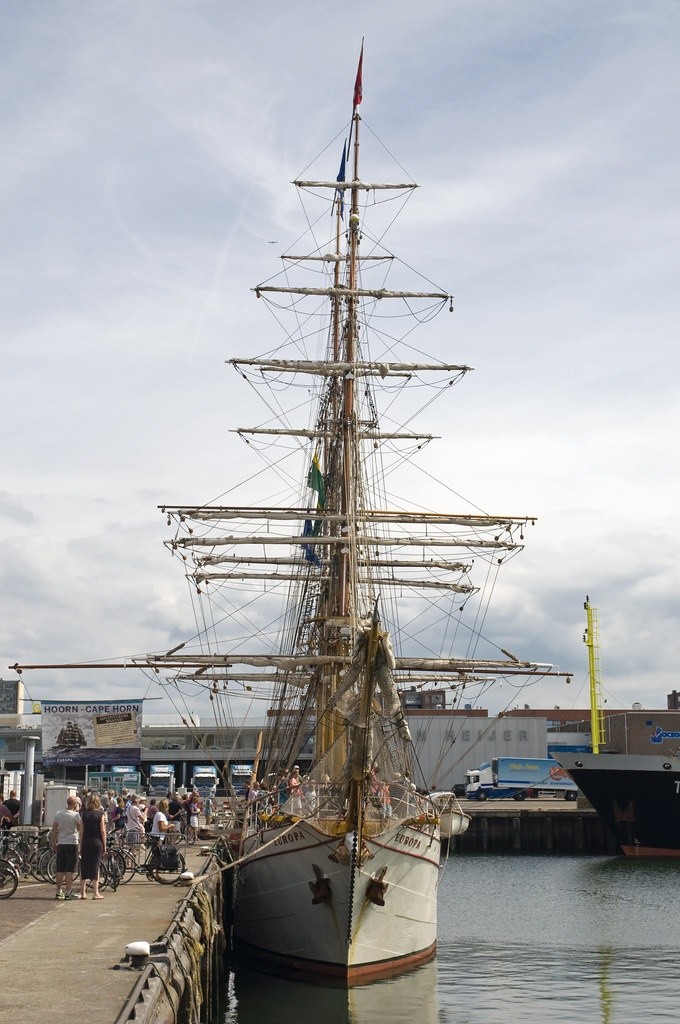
[208,794,215,798]
[294,765,299,768]
[140,796,147,801]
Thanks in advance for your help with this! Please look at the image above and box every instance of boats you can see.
[548,750,680,859]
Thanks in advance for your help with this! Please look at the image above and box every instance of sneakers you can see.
[55,892,65,899]
[65,891,78,900]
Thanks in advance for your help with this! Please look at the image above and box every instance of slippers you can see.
[92,894,104,900]
[81,896,88,899]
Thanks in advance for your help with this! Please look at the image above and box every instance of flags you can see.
[307,454,326,535]
[301,520,322,568]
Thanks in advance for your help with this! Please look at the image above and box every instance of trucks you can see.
[233,766,254,791]
[146,764,176,798]
[464,756,578,799]
[191,766,216,792]
[112,764,135,774]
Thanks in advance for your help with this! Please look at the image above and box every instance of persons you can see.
[0,792,17,826]
[146,799,176,882]
[205,794,216,825]
[79,794,107,900]
[2,790,20,830]
[51,796,84,899]
[76,787,203,850]
[240,765,331,816]
[369,761,416,814]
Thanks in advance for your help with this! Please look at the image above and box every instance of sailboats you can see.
[130,36,573,990]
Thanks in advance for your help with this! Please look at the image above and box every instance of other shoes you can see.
[146,874,155,881]
[155,877,168,881]
[195,838,200,841]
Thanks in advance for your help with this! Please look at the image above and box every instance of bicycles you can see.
[0,828,187,899]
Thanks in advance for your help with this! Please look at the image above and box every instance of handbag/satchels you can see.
[161,835,180,870]
